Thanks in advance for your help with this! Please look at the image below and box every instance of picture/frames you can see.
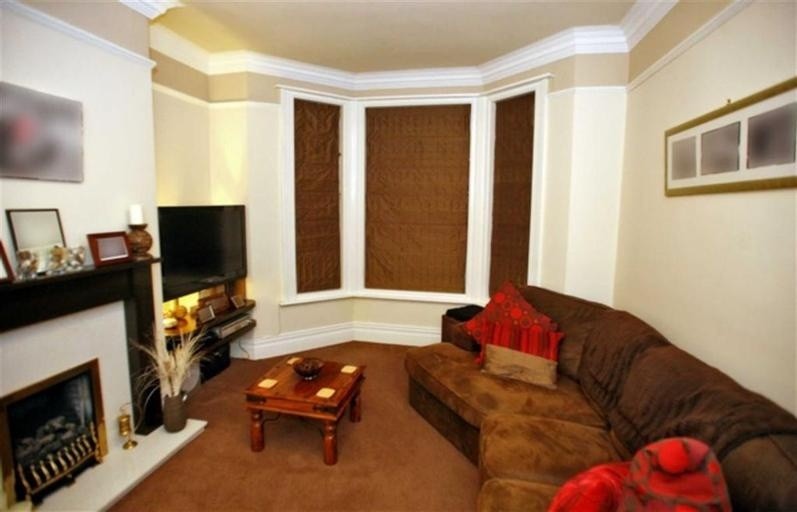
[664,75,797,197]
[0,241,15,284]
[86,231,134,268]
[5,208,66,276]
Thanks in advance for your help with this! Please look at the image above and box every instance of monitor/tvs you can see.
[158,205,247,302]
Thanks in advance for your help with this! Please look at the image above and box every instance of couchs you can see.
[404,279,797,512]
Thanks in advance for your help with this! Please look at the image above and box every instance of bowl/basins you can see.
[292,356,325,382]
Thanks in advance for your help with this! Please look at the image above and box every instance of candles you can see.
[130,203,143,224]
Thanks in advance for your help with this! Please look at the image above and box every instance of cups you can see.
[14,245,85,280]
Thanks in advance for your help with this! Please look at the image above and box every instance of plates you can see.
[163,316,177,330]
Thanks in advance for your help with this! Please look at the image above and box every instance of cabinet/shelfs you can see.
[164,273,256,385]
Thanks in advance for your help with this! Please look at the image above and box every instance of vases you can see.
[163,392,187,432]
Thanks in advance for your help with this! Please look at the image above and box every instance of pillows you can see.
[617,436,733,512]
[474,303,567,365]
[463,280,559,345]
[547,459,633,512]
[480,343,559,390]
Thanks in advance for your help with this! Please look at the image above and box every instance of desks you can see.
[442,315,479,352]
